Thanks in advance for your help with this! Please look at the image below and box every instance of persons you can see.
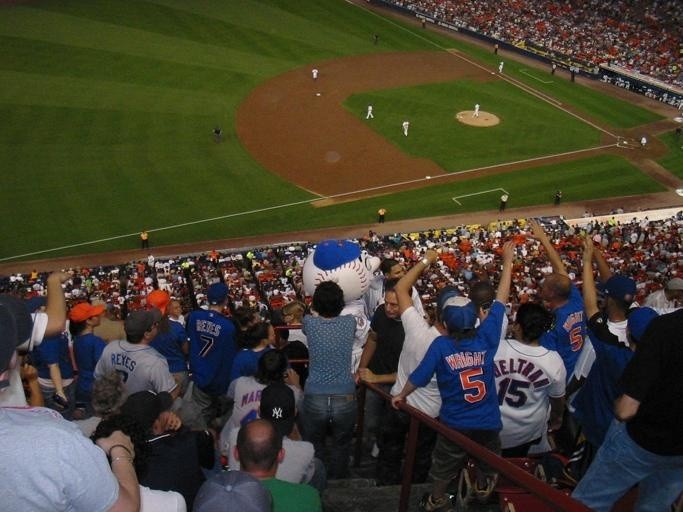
[366,104,375,120]
[498,193,508,212]
[472,103,480,119]
[402,119,409,137]
[553,190,562,206]
[640,136,647,147]
[312,67,319,82]
[387,0,683,112]
[213,126,221,144]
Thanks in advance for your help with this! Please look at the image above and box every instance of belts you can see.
[304,393,359,401]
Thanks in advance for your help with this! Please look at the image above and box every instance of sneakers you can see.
[418,491,455,512]
[472,472,499,506]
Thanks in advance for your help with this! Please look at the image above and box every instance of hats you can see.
[436,285,477,330]
[626,307,660,342]
[595,273,637,305]
[118,390,174,429]
[0,293,35,373]
[664,277,683,292]
[207,283,229,305]
[191,470,273,512]
[145,289,169,315]
[259,382,296,436]
[68,301,105,323]
[613,242,621,249]
[592,234,603,242]
